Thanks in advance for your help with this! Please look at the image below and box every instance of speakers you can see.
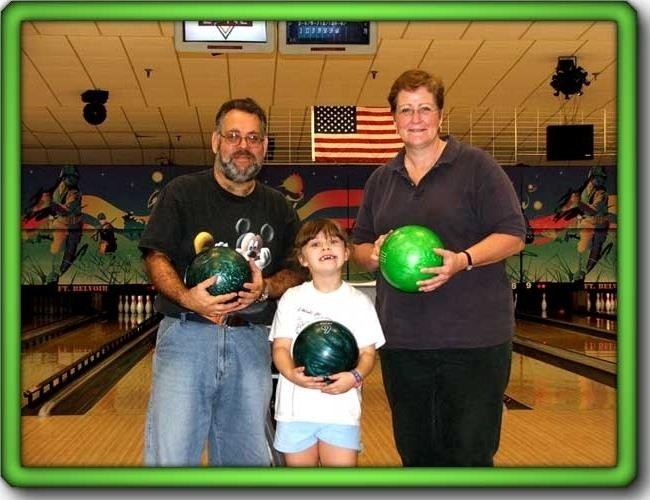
[546,124,594,161]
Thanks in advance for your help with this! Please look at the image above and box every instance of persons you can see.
[138,97,309,467]
[268,217,387,467]
[350,69,527,467]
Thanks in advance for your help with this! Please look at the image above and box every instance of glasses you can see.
[220,133,264,145]
[398,106,440,115]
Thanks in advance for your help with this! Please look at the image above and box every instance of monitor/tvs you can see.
[173,20,276,56]
[278,20,378,56]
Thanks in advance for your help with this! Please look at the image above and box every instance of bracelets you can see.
[349,369,361,388]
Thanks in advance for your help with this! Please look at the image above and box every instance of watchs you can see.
[458,250,473,272]
[254,280,268,305]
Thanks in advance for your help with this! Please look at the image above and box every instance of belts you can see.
[166,312,273,326]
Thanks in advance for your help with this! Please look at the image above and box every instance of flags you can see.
[311,105,441,163]
